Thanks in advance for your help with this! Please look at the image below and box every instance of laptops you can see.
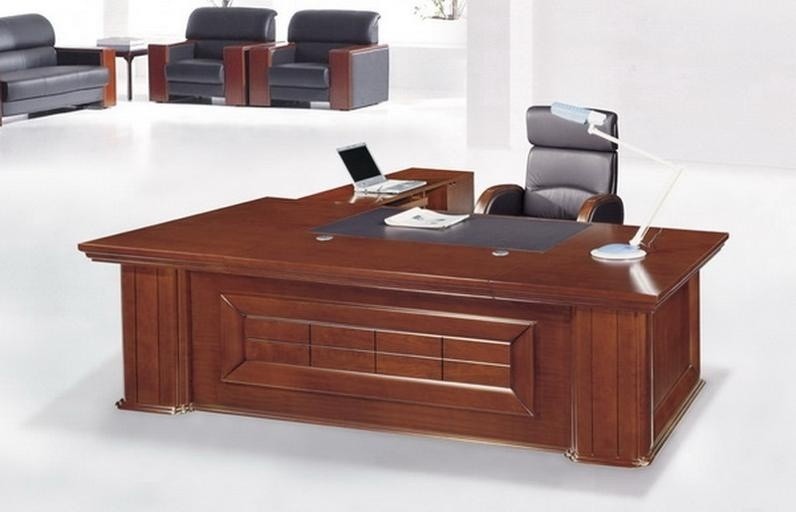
[349,193,396,205]
[337,141,429,194]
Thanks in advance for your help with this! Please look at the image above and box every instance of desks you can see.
[77,165,732,470]
[114,49,149,102]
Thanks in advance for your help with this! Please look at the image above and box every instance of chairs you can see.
[470,104,626,223]
[143,4,391,111]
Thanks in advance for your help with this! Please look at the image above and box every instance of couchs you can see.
[0,13,116,128]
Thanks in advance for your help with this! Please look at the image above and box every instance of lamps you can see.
[549,102,687,267]
